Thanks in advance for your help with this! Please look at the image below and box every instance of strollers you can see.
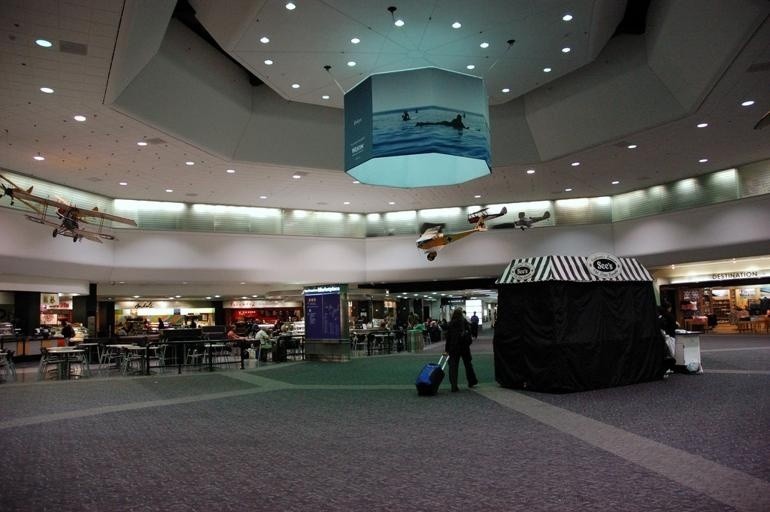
[470,326,480,342]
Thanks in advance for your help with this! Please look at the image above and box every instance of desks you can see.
[684,317,709,331]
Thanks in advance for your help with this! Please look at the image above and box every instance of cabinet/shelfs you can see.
[712,297,730,324]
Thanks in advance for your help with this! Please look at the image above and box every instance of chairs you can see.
[0,325,431,381]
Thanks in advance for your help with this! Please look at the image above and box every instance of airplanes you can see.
[489,209,551,234]
[468,205,508,224]
[413,214,489,263]
[0,174,46,216]
[11,189,137,246]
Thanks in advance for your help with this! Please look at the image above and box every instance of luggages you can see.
[416,354,450,395]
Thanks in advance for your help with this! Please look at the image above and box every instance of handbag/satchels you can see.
[460,330,472,347]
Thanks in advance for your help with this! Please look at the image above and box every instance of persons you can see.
[660,298,681,357]
[227,315,302,362]
[357,311,448,353]
[117,316,196,337]
[445,309,477,392]
[415,114,470,128]
[64,208,80,229]
[402,110,410,121]
[470,312,479,338]
[61,321,74,347]
